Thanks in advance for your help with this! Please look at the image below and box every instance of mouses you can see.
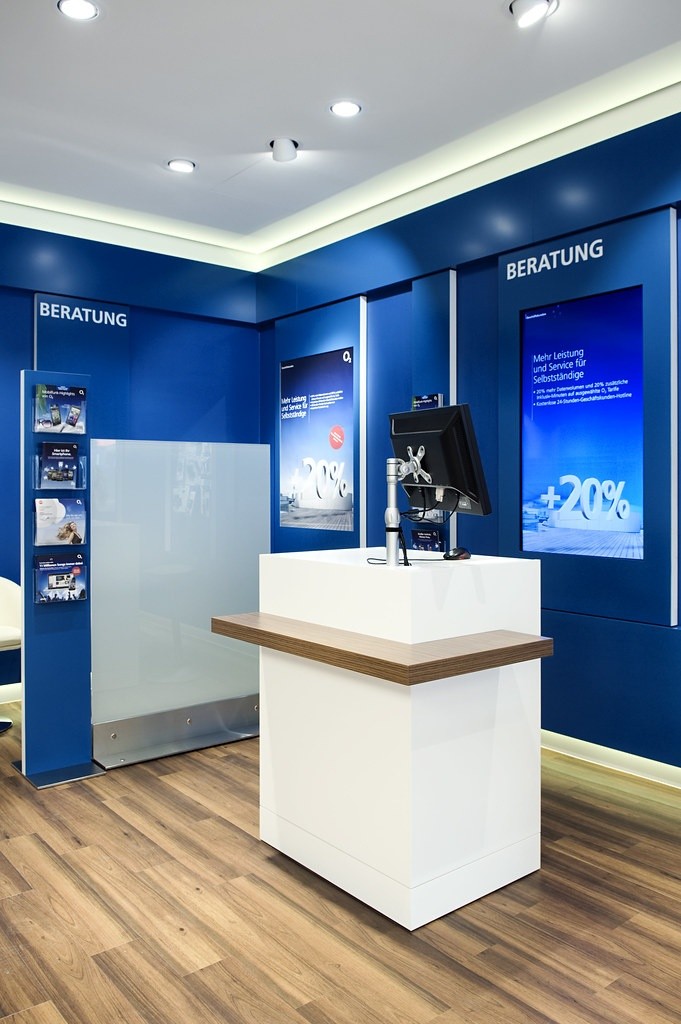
[443,547,471,560]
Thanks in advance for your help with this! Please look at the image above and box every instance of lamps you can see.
[512,0,549,28]
[272,140,298,162]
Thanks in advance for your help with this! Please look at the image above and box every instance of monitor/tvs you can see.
[388,404,493,516]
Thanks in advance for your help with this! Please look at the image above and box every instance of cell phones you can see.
[51,407,61,425]
[66,405,82,427]
